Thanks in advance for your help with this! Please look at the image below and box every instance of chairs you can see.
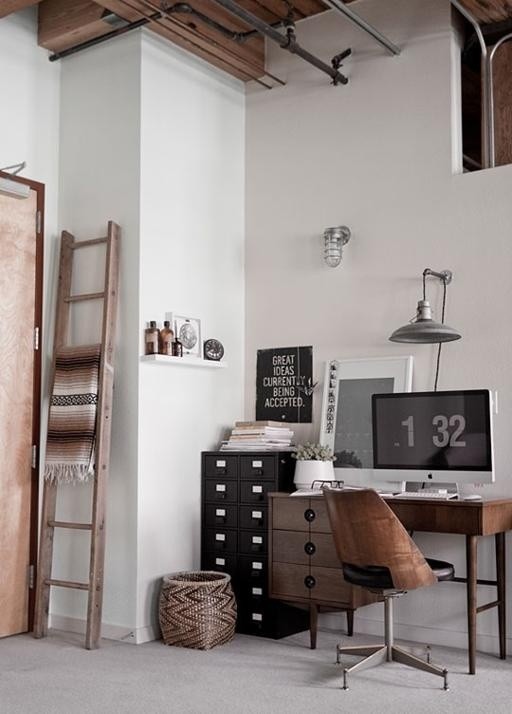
[323,488,455,691]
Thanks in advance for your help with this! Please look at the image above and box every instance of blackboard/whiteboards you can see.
[256,346,313,423]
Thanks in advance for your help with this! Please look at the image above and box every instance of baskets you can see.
[157,570,238,652]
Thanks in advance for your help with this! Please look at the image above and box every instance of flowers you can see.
[334,450,362,468]
[291,442,338,462]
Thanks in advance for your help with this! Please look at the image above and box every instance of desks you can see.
[267,492,512,674]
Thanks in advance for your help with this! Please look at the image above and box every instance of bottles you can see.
[144,320,182,356]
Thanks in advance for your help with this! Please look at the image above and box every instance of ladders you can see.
[33,220,121,650]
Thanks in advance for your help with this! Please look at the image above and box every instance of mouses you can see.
[464,495,481,501]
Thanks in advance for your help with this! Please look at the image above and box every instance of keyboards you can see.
[393,492,458,500]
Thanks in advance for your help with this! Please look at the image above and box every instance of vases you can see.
[293,460,336,490]
[336,464,355,467]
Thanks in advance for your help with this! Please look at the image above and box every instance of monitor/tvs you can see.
[371,389,495,500]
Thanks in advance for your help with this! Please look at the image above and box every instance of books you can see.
[218,418,295,451]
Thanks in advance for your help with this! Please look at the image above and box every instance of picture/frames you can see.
[167,312,204,359]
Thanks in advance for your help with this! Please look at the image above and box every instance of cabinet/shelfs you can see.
[201,451,311,640]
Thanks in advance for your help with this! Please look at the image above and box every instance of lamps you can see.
[324,226,350,268]
[389,269,462,344]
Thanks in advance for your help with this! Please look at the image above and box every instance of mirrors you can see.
[320,356,413,493]
